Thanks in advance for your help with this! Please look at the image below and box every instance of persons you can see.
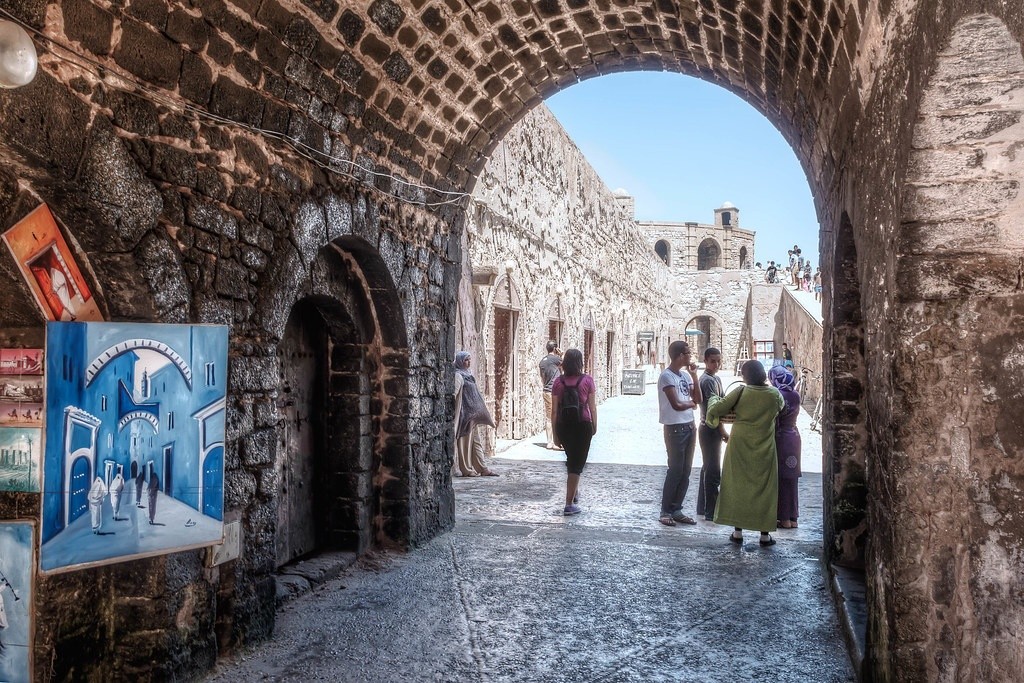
[705,360,785,547]
[788,244,822,303]
[550,349,598,515]
[658,340,704,526]
[768,365,802,529]
[754,262,763,271]
[453,352,503,476]
[699,348,729,521]
[781,343,797,377]
[538,340,564,451]
[765,261,778,283]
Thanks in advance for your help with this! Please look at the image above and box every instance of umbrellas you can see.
[685,329,704,346]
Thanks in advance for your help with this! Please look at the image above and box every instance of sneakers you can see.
[564,505,582,516]
[573,495,580,504]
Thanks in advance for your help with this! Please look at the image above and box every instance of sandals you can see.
[659,513,677,527]
[674,514,697,526]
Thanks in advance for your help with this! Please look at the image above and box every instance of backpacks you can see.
[558,374,587,426]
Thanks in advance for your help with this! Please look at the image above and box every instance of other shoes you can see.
[759,535,777,548]
[729,532,744,544]
[777,517,793,529]
[547,443,553,448]
[791,521,798,528]
[481,470,501,476]
[552,444,565,451]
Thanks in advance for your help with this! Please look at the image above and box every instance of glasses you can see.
[681,351,691,354]
